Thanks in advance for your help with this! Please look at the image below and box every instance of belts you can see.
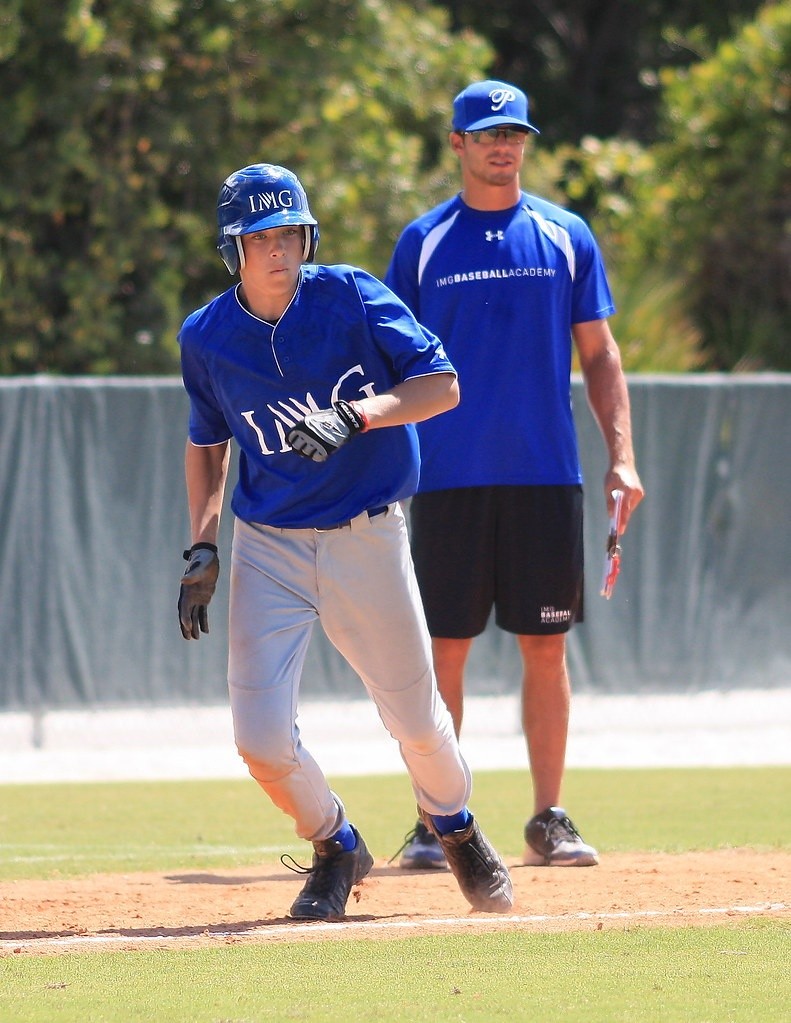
[314,506,389,532]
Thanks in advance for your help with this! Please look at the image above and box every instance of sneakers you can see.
[388,818,447,869]
[417,801,513,913]
[280,823,373,923]
[522,806,600,866]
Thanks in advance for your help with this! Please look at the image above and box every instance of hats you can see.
[452,78,540,137]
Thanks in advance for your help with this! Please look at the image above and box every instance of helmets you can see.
[216,164,319,275]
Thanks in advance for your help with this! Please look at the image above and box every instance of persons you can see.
[386,80,646,868]
[176,163,514,922]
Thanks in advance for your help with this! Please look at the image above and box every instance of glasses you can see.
[460,128,527,145]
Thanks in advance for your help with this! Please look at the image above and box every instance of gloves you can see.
[285,399,366,462]
[177,542,220,640]
